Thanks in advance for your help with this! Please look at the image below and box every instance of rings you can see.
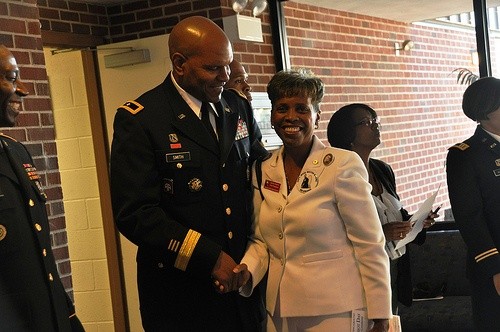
[400,232,403,238]
[430,222,433,226]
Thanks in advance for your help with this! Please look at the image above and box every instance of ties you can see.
[201,103,219,144]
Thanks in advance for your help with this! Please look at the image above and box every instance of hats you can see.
[462,77,500,119]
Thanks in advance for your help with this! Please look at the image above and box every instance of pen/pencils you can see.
[433,202,444,214]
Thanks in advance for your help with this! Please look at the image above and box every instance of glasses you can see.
[352,117,379,129]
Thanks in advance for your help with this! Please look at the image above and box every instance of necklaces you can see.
[283,151,311,193]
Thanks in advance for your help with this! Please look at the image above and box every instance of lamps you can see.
[394,40,413,57]
[223,0,268,46]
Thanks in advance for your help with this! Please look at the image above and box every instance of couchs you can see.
[411,217,468,295]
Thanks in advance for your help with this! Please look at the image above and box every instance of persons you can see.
[222,58,255,106]
[106,16,272,332]
[325,103,440,332]
[208,66,393,332]
[0,45,88,331]
[446,76,500,332]
